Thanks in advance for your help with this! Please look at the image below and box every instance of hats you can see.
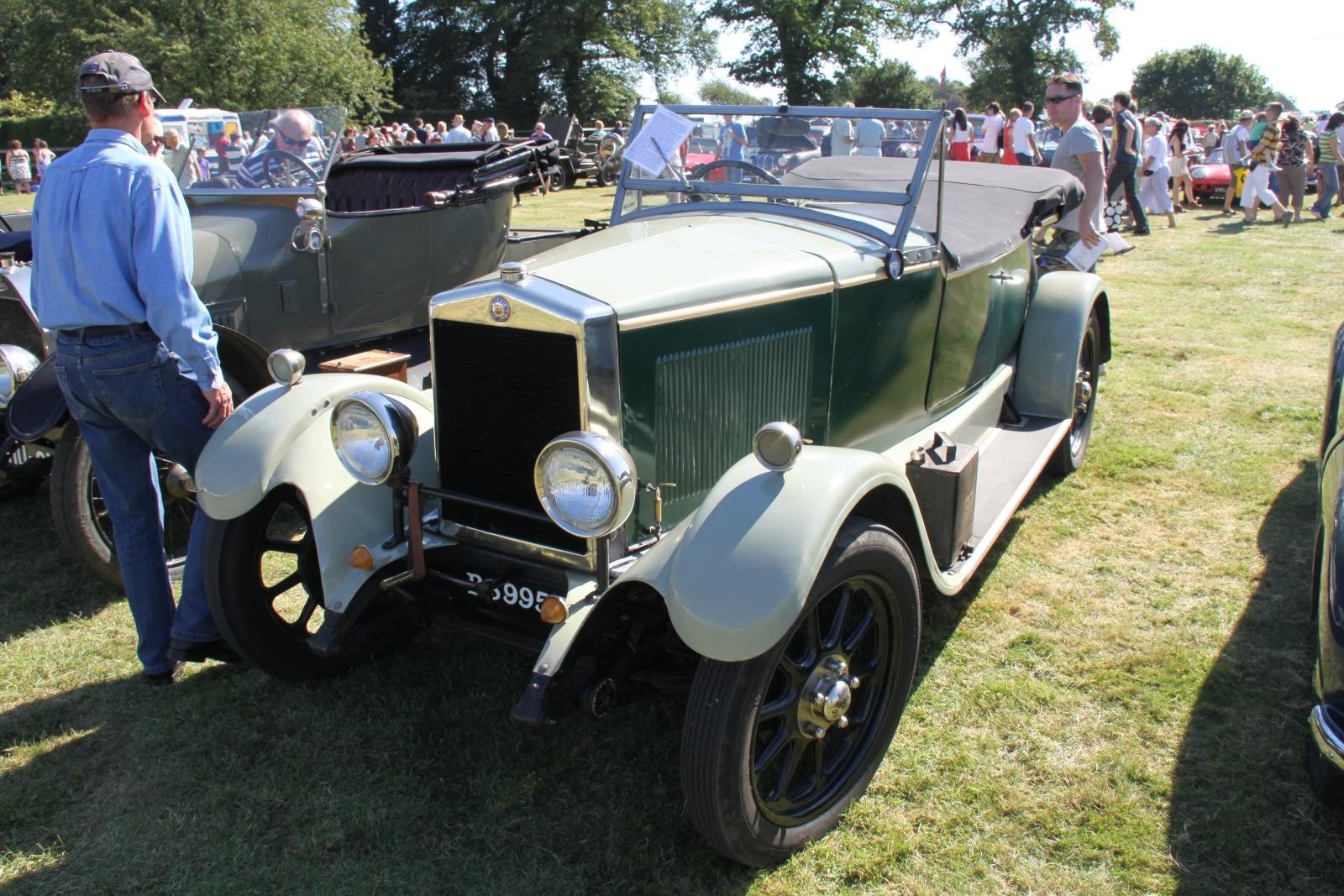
[77,49,168,104]
[482,118,494,122]
[1241,110,1254,120]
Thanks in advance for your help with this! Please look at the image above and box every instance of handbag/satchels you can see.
[1223,127,1243,164]
[1313,137,1320,164]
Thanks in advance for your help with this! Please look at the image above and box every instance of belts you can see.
[58,322,149,337]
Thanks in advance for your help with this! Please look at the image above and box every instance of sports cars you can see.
[1183,148,1235,203]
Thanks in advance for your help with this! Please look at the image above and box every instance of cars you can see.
[895,113,1204,193]
[520,104,860,191]
[1307,320,1344,778]
[177,100,1111,864]
[0,103,594,593]
[1303,130,1320,192]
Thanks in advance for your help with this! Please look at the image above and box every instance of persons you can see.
[1035,73,1106,375]
[948,91,1202,246]
[0,138,56,195]
[530,122,553,195]
[714,114,748,201]
[213,109,329,189]
[592,120,606,136]
[340,115,521,208]
[570,118,584,139]
[29,50,244,685]
[1204,101,1344,228]
[663,136,689,204]
[830,102,927,157]
[611,120,623,135]
[163,128,210,189]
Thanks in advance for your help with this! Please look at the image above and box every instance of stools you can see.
[320,351,411,386]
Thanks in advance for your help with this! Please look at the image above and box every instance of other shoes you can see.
[146,660,181,686]
[1268,165,1283,172]
[530,189,539,195]
[1234,220,1255,229]
[167,638,245,664]
[1283,211,1294,227]
[513,203,522,208]
[1222,207,1236,215]
[1309,207,1322,218]
[1134,227,1150,234]
[1239,158,1248,167]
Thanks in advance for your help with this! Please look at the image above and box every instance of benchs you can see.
[324,167,471,214]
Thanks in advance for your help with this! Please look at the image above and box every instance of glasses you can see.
[278,132,311,148]
[1044,94,1077,104]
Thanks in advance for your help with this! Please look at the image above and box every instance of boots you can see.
[1188,201,1202,210]
[1173,205,1186,213]
